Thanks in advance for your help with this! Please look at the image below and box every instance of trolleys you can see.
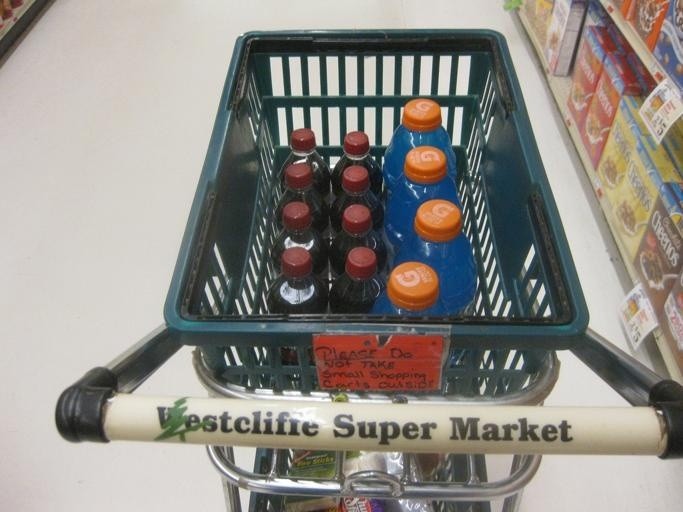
[54,23,682,511]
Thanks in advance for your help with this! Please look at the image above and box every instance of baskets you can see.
[248,449,494,512]
[163,28,589,398]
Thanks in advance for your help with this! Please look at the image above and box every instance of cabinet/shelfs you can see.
[503,0,682,411]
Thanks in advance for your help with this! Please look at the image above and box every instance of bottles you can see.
[370,97,481,390]
[332,130,386,389]
[265,127,332,390]
